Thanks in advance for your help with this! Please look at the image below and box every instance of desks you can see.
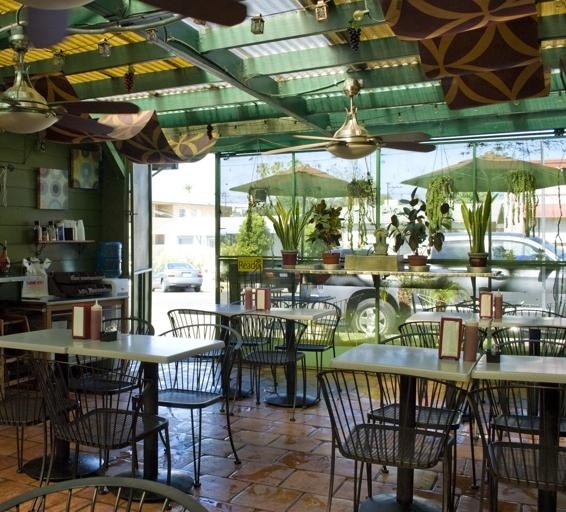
[264,266,500,344]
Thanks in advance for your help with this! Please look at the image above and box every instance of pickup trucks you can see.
[313,227,566,338]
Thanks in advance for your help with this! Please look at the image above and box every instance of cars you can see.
[151,260,205,294]
[223,242,373,294]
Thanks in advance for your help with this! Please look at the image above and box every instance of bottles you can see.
[494,287,505,320]
[491,343,498,354]
[465,320,477,363]
[89,299,103,341]
[244,283,253,309]
[33,219,86,241]
[104,326,115,332]
[1,240,10,274]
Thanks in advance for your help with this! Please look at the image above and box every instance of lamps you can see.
[251,13,265,35]
[145,28,159,44]
[53,50,66,67]
[351,8,370,23]
[314,1,328,22]
[98,39,112,57]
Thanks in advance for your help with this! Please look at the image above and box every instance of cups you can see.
[316,285,325,298]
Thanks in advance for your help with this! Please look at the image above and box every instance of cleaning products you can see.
[72,219,86,241]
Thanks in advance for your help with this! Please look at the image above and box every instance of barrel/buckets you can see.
[95,242,122,277]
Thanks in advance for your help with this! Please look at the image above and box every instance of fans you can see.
[260,77,436,159]
[15,0,250,27]
[0,34,142,135]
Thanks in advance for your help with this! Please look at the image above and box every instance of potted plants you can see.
[460,189,498,266]
[264,194,315,264]
[305,199,345,263]
[386,186,454,265]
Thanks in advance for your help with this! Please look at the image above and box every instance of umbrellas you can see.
[228,164,374,259]
[400,152,566,291]
